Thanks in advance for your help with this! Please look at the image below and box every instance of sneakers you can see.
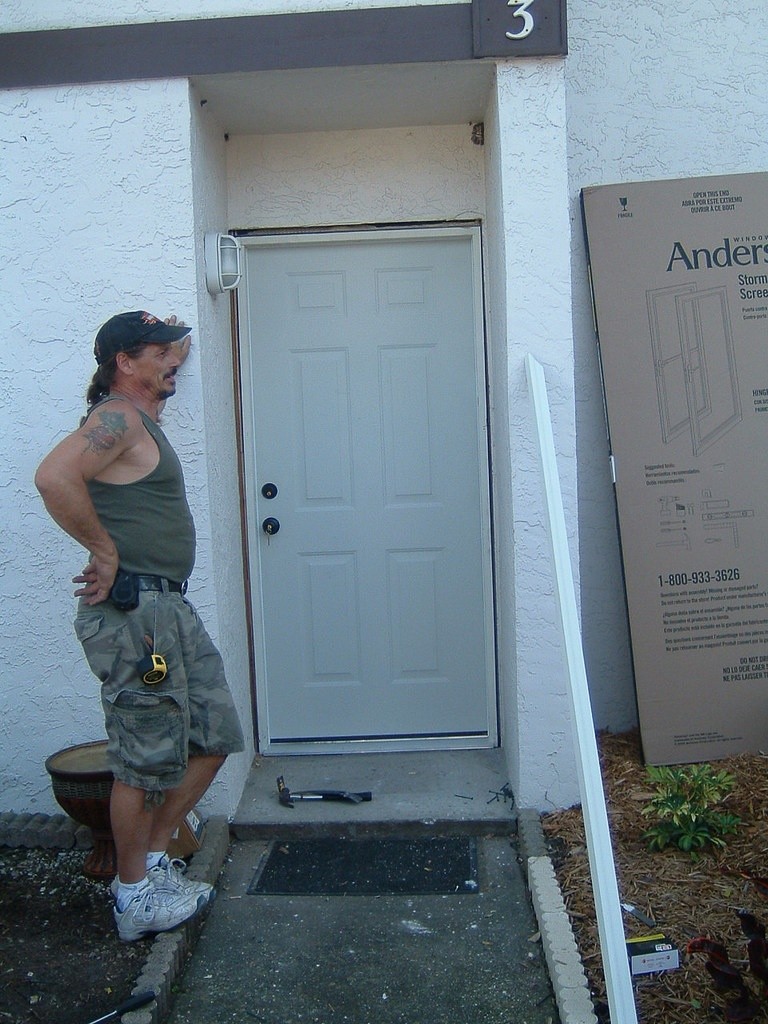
[109,852,217,903]
[113,881,208,943]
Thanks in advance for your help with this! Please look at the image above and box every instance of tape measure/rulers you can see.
[138,654,167,687]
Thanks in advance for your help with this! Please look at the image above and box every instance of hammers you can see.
[277,786,373,809]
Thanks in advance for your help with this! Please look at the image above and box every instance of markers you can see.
[145,633,154,650]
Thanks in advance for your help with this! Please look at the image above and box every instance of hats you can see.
[94,310,193,364]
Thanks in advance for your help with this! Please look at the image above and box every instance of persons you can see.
[34,312,245,942]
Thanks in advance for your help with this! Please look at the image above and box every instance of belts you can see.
[137,575,188,597]
